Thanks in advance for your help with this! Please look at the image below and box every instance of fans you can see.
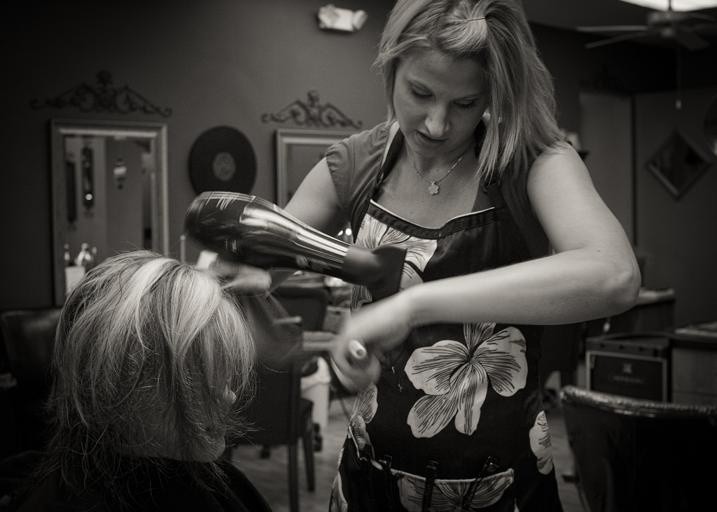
[564,1,716,55]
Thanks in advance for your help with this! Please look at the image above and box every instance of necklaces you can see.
[403,133,480,197]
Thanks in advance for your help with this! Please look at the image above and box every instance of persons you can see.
[41,248,274,510]
[199,0,641,511]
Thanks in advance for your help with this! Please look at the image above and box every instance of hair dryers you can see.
[182,189,410,304]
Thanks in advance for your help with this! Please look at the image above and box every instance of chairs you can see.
[555,378,717,510]
[220,288,324,511]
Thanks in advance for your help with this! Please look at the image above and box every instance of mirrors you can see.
[274,125,358,214]
[46,116,172,310]
